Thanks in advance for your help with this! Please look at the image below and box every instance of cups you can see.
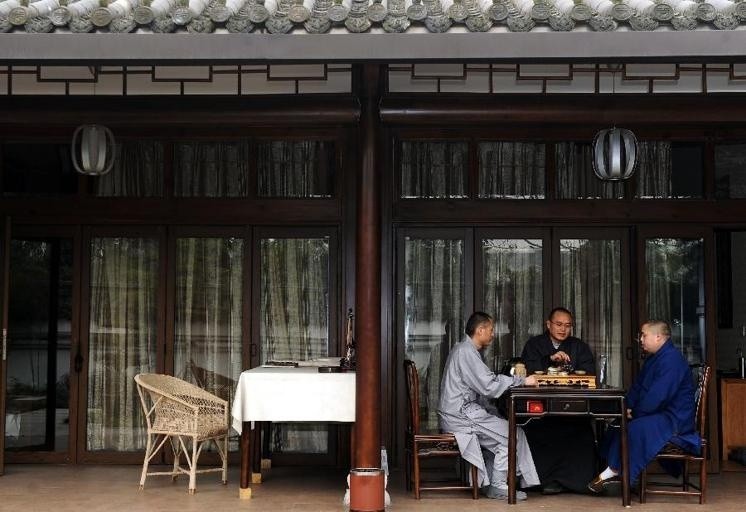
[534,362,587,376]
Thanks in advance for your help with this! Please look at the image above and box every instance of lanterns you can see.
[69,124,117,179]
[586,124,642,186]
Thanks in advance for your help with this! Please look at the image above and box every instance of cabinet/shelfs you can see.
[722,374,746,461]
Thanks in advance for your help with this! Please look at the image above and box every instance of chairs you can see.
[563,417,602,478]
[132,374,230,494]
[403,359,479,500]
[638,363,712,504]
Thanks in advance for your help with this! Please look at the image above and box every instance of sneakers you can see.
[602,481,622,492]
[543,482,563,493]
[482,486,527,500]
[588,473,620,492]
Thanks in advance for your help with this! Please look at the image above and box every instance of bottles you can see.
[501,359,527,377]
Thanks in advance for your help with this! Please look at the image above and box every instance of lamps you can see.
[590,64,640,182]
[69,60,117,175]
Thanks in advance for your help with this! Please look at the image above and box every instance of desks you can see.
[239,365,356,501]
[491,381,631,508]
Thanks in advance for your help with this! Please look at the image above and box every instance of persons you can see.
[520,305,605,497]
[496,311,539,364]
[435,310,541,503]
[585,316,703,497]
[424,316,504,431]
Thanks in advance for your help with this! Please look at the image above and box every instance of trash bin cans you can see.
[350,468,385,512]
